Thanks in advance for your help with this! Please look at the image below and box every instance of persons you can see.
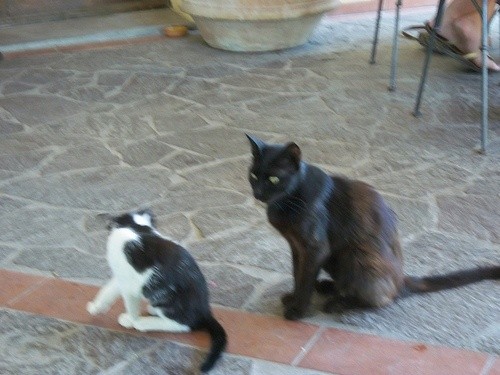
[425,0,500,72]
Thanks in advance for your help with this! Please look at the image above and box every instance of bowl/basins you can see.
[175,0,342,53]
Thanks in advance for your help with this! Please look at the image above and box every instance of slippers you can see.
[419,22,495,73]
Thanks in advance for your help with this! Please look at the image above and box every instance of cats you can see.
[84,210,229,374]
[245,129,498,322]
